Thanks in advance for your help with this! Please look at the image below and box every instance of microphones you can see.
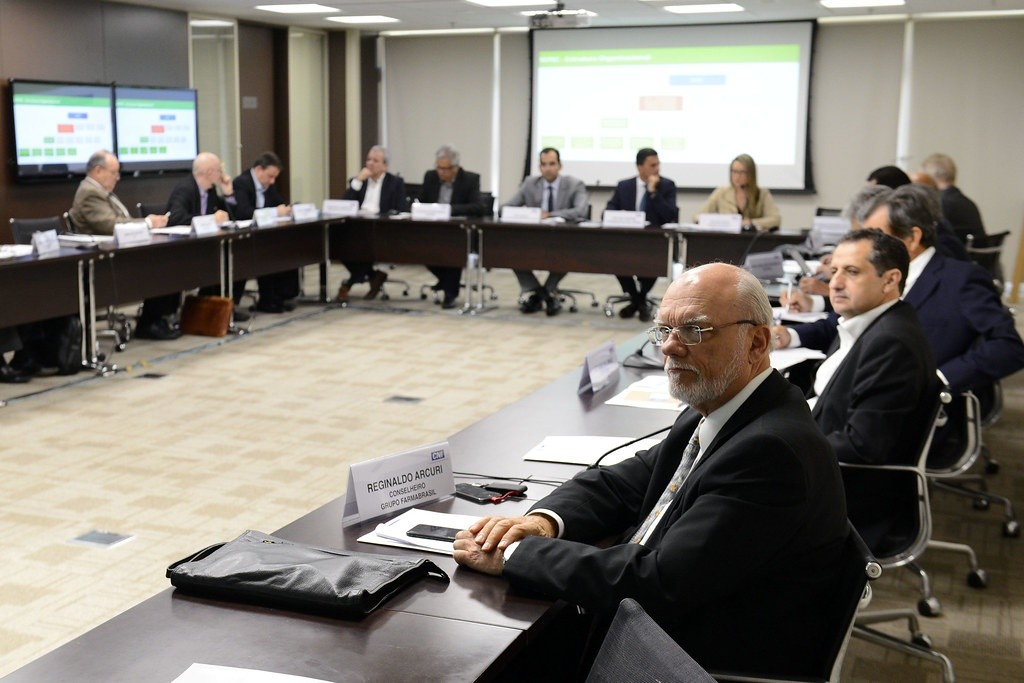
[390,173,400,215]
[575,181,601,226]
[738,226,780,267]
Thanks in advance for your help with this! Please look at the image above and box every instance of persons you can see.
[693,154,781,230]
[231,153,288,312]
[498,147,587,316]
[165,151,248,320]
[773,154,1024,452]
[453,263,847,683]
[0,327,43,382]
[72,150,182,338]
[420,146,484,308]
[602,148,677,320]
[339,146,405,300]
[814,228,935,547]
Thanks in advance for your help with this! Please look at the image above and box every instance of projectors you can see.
[529,12,591,28]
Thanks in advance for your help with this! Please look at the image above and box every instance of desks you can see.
[0,205,837,683]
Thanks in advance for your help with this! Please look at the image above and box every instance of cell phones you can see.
[453,483,503,504]
[406,524,465,542]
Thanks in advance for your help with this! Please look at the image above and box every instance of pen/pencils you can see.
[785,280,793,314]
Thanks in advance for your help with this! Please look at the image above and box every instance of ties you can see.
[639,184,650,211]
[629,420,705,545]
[547,185,553,213]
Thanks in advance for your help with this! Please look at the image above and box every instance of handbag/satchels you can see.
[14,316,82,376]
[166,528,450,621]
[179,295,233,337]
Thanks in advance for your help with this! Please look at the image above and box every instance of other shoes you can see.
[637,302,650,322]
[619,304,636,318]
[132,317,181,340]
[546,296,560,316]
[233,303,251,322]
[519,296,542,314]
[0,356,31,384]
[442,286,460,309]
[363,270,387,299]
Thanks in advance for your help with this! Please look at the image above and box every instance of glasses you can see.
[647,319,759,347]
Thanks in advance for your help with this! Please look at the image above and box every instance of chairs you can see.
[134,203,167,218]
[362,182,678,317]
[8,215,122,365]
[708,229,1014,683]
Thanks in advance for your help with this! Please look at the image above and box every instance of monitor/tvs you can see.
[6,77,200,184]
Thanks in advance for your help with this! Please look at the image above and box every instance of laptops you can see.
[790,248,814,278]
